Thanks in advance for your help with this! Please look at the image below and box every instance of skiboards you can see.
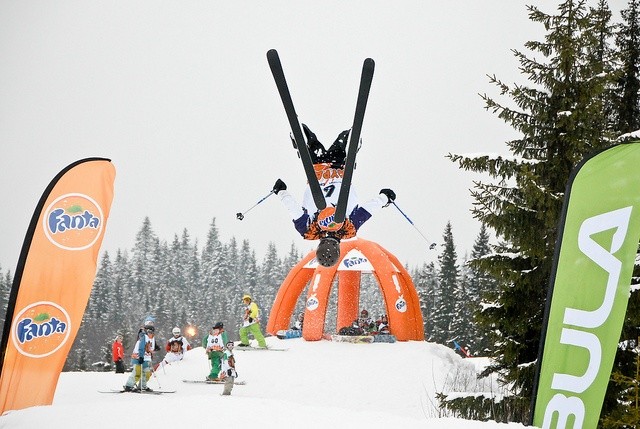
[98,388,177,395]
[182,379,248,385]
[266,49,375,223]
[234,345,291,352]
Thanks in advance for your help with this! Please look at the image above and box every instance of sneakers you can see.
[125,385,134,391]
[138,386,153,392]
[238,343,250,347]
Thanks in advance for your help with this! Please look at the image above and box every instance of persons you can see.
[273,123,396,267]
[124,325,156,392]
[202,322,229,381]
[358,310,374,328]
[221,340,238,395]
[238,295,266,347]
[379,314,389,334]
[291,312,304,330]
[165,327,191,353]
[465,347,472,358]
[162,340,183,364]
[113,335,125,373]
[137,317,161,390]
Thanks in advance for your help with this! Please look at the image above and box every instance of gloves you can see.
[270,179,287,195]
[379,188,396,208]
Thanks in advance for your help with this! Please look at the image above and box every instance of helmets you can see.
[316,238,340,267]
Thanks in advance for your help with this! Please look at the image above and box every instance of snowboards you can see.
[331,335,375,344]
[372,335,396,343]
